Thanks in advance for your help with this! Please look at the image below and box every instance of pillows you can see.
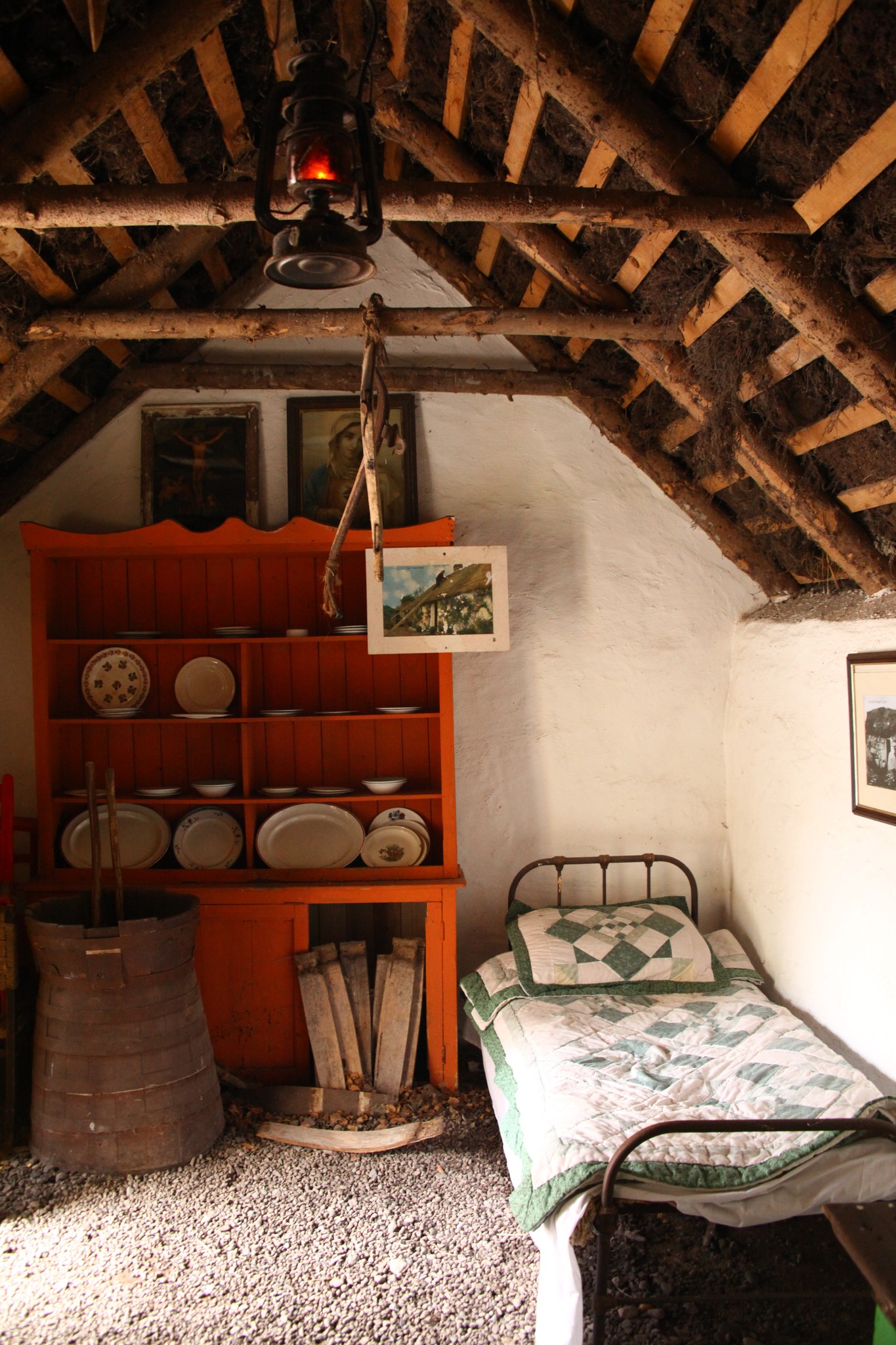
[504,896,730,996]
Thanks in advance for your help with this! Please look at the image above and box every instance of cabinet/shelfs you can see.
[19,515,457,882]
[160,888,458,1093]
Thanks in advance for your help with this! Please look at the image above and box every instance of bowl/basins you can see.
[286,629,308,637]
[361,777,408,794]
[190,781,237,797]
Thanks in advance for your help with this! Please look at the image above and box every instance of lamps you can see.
[254,0,385,291]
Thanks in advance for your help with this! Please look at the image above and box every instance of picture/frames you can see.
[287,393,419,530]
[846,652,896,826]
[141,401,263,533]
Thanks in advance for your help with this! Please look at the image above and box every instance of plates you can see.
[256,803,365,868]
[333,625,368,635]
[260,710,304,717]
[82,646,150,718]
[213,627,260,639]
[375,707,420,713]
[135,786,180,798]
[257,785,304,797]
[175,807,243,868]
[65,789,116,798]
[60,803,171,870]
[116,631,161,640]
[361,806,430,867]
[314,711,359,715]
[308,786,354,796]
[171,657,236,719]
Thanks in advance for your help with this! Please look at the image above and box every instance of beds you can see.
[457,854,896,1345]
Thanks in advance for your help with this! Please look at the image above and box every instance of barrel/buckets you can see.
[27,888,224,1178]
